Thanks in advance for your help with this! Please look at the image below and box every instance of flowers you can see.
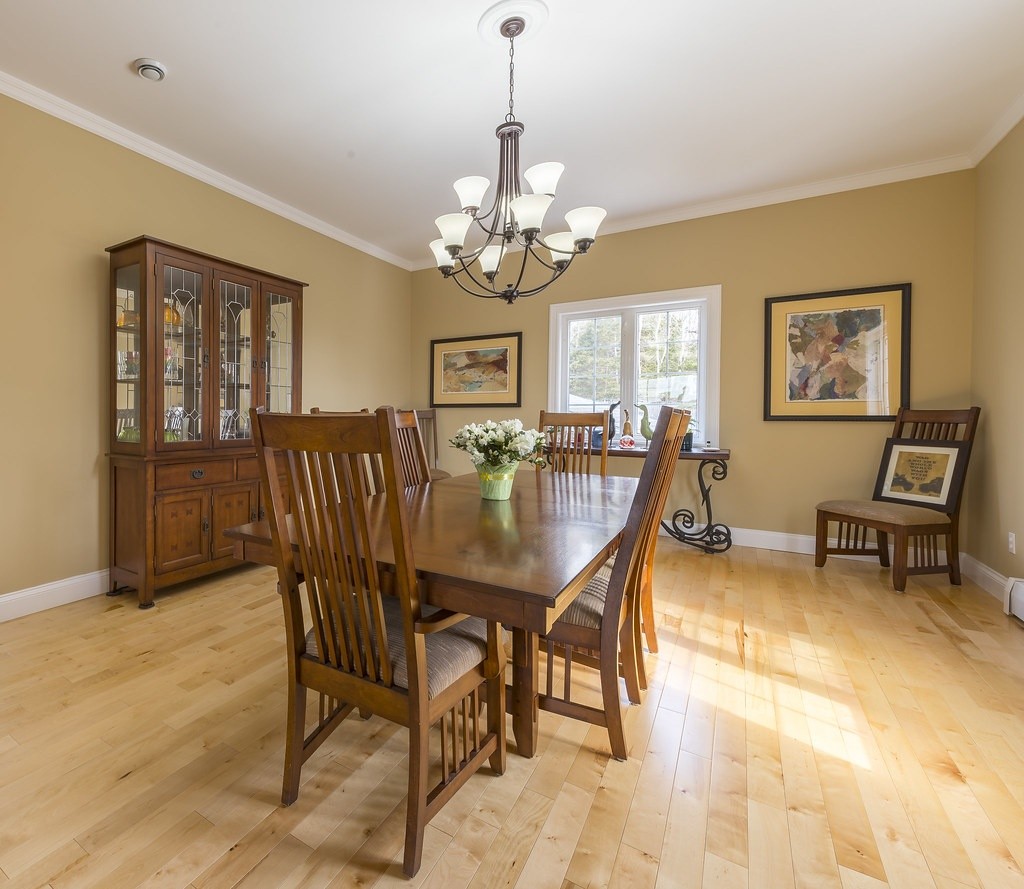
[449,418,546,469]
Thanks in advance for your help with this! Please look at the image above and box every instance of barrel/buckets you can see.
[680,433,693,450]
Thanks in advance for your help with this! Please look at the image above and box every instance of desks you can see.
[221,470,640,759]
[543,444,732,554]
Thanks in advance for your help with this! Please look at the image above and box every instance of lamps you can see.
[428,34,607,306]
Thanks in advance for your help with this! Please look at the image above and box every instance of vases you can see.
[470,459,519,501]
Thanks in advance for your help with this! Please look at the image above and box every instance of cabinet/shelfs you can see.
[103,234,310,610]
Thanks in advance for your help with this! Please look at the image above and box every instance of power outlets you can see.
[1008,531,1016,554]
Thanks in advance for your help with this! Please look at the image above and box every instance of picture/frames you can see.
[430,331,522,407]
[871,438,971,514]
[762,283,913,423]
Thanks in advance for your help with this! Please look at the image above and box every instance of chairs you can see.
[397,409,451,484]
[499,404,683,762]
[814,406,982,591]
[535,410,610,475]
[552,410,692,691]
[247,406,507,880]
[373,409,432,488]
[309,407,386,611]
